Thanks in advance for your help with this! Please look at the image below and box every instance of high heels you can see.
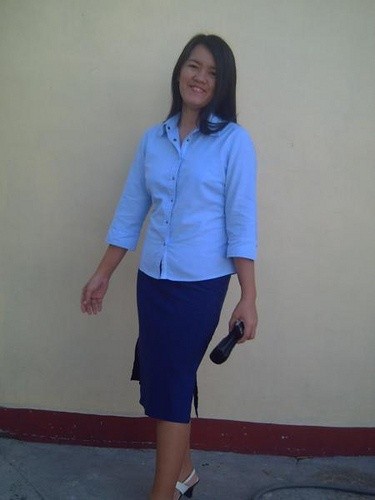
[170,469,201,500]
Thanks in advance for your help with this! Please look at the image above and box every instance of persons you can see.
[78,33,259,499]
